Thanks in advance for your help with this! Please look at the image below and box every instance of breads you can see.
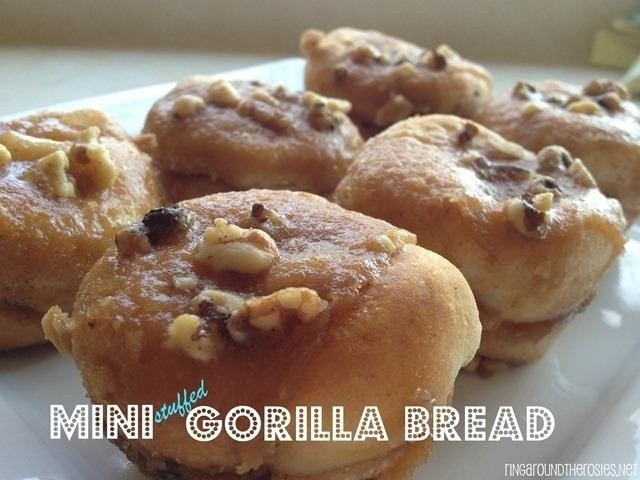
[0,26,640,480]
